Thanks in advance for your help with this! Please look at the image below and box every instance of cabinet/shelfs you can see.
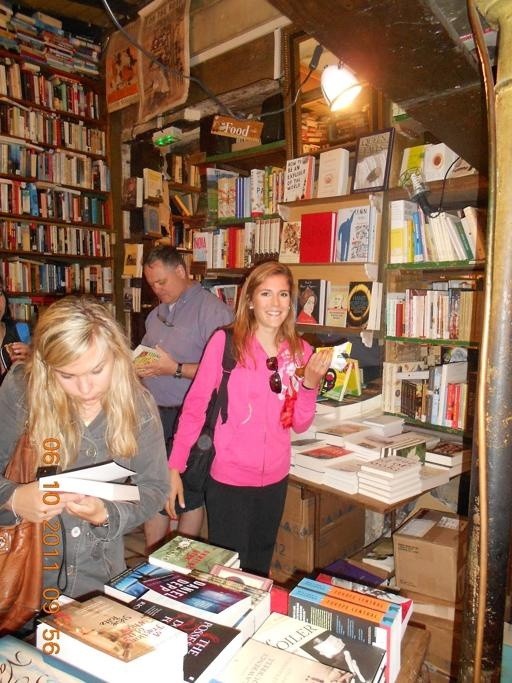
[0,36,490,683]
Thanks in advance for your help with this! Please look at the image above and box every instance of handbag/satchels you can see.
[158,430,216,515]
[0,433,43,637]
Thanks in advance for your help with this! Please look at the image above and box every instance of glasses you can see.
[157,298,177,328]
[266,357,281,393]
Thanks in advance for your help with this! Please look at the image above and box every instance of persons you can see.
[121,243,236,558]
[0,296,173,639]
[162,261,335,579]
[0,290,30,381]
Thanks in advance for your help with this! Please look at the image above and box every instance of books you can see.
[37,460,144,504]
